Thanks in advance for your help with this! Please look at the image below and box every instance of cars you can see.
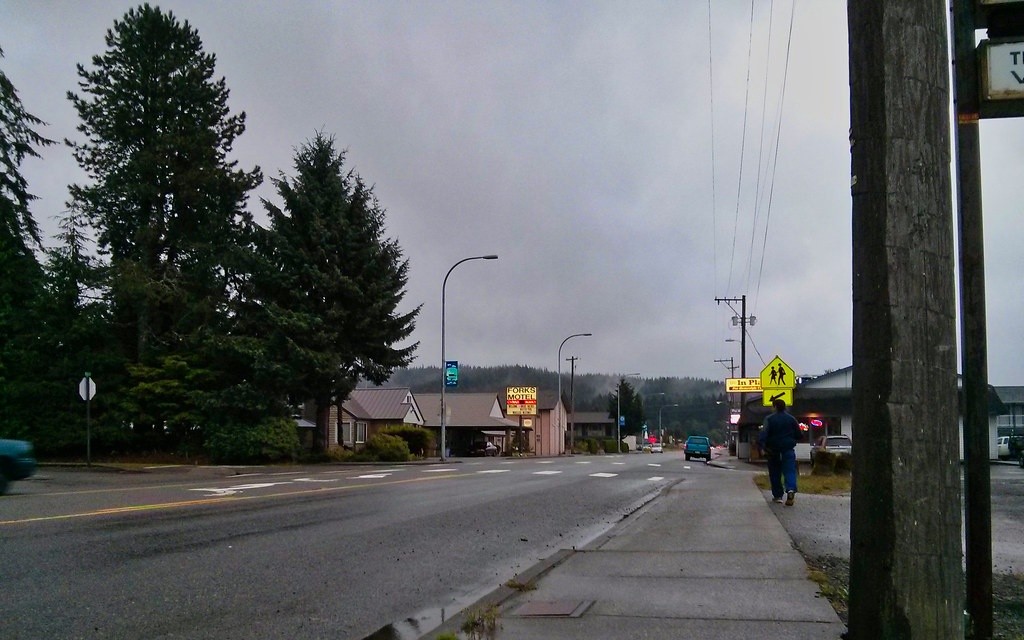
[643,444,654,452]
[651,444,663,453]
[683,436,711,461]
[1,440,35,493]
[1018,450,1024,468]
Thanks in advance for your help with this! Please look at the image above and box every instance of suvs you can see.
[810,434,852,467]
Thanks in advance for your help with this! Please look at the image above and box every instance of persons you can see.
[758,399,800,506]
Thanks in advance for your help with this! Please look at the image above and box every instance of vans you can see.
[997,436,1024,460]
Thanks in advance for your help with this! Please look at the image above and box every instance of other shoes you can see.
[772,496,783,503]
[785,490,795,506]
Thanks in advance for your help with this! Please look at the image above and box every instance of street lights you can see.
[659,404,678,437]
[441,255,498,456]
[558,334,593,453]
[618,372,640,452]
